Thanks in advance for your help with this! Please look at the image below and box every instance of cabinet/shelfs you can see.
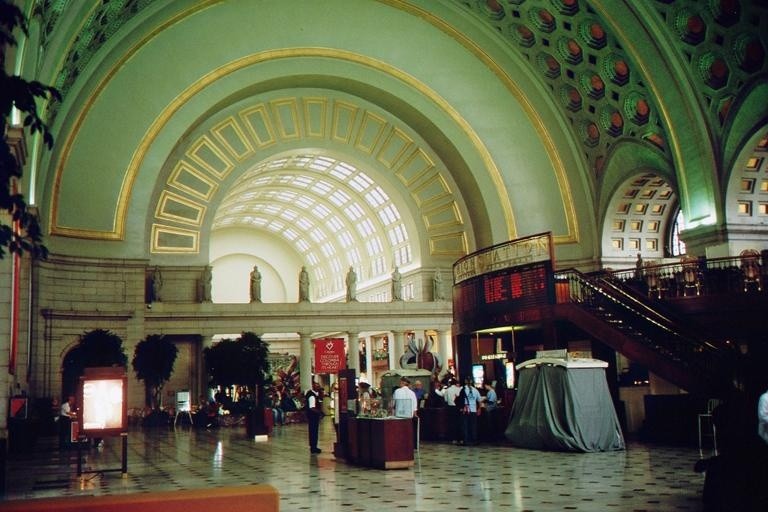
[348,417,414,470]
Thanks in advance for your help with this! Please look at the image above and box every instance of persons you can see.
[270,392,285,426]
[358,383,371,414]
[298,265,309,303]
[411,380,426,442]
[305,383,324,454]
[344,265,358,304]
[431,268,446,302]
[201,264,213,302]
[59,395,76,465]
[756,388,768,446]
[390,376,418,419]
[152,264,164,300]
[434,376,498,447]
[390,266,402,303]
[249,264,262,303]
